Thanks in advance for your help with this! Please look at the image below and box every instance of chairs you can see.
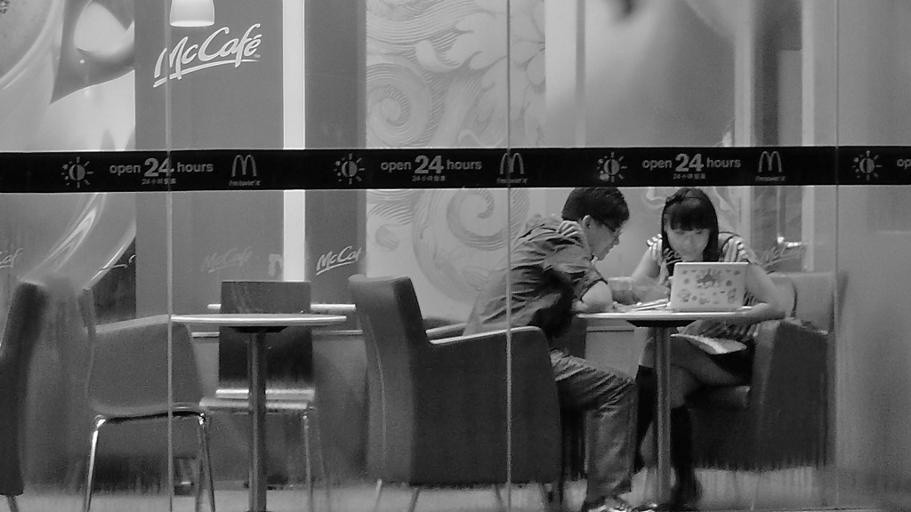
[76,285,218,511]
[344,273,563,512]
[0,281,48,512]
[641,273,848,511]
[196,279,333,511]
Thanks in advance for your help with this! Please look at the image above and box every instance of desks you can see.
[168,312,350,511]
[574,302,751,512]
[206,303,356,315]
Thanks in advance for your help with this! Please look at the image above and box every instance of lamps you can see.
[169,0,215,28]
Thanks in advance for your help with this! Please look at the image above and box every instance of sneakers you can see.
[582,495,634,512]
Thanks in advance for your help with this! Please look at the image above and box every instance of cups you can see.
[608,277,632,309]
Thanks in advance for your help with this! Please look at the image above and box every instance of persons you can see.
[630,185,789,512]
[460,186,640,512]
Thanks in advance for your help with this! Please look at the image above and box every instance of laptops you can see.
[665,260,752,314]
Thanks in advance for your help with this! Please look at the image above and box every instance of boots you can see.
[635,365,702,511]
